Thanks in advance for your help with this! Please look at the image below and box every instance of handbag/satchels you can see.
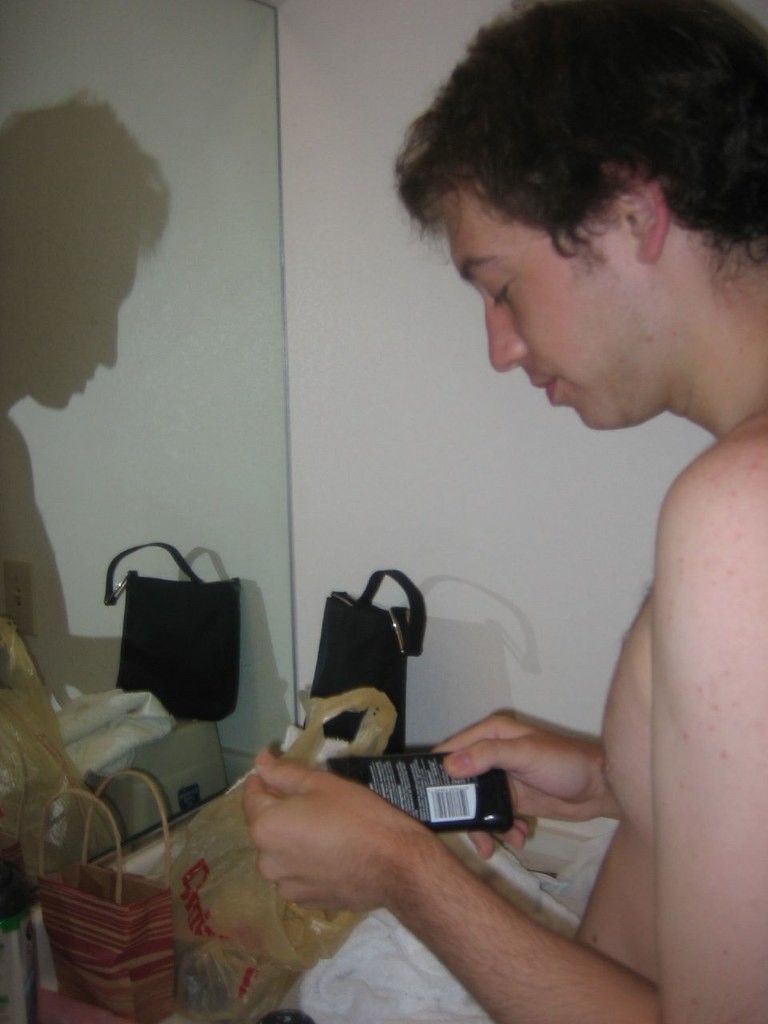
[36,769,174,1023]
[303,570,428,755]
[146,686,400,1022]
[0,616,114,880]
[103,542,242,721]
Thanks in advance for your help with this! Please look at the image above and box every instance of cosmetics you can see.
[325,750,517,834]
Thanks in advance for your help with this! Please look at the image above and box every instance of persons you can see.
[241,3,765,1019]
